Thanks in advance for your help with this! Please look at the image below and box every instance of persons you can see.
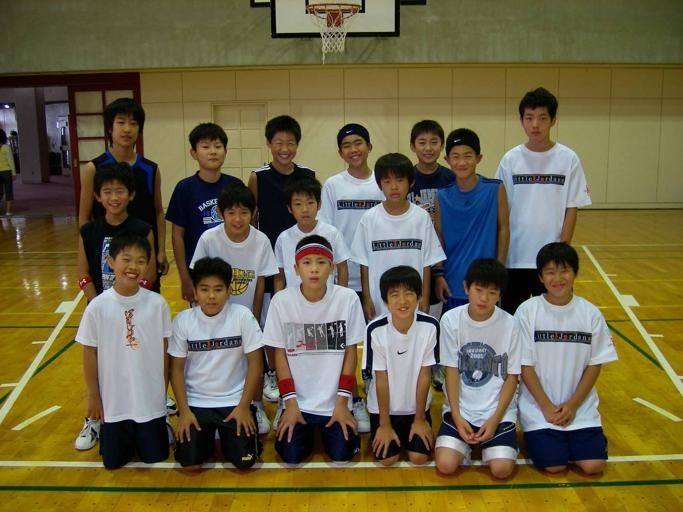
[248,115,316,401]
[79,97,178,416]
[263,235,367,464]
[74,161,156,451]
[188,183,280,435]
[0,128,17,215]
[166,257,266,471]
[272,172,352,432]
[165,123,245,308]
[74,232,175,471]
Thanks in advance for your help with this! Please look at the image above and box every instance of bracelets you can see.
[276,378,296,401]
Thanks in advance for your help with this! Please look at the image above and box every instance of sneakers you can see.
[249,399,282,434]
[263,369,281,400]
[432,364,446,387]
[74,415,100,451]
[352,398,371,433]
[166,420,176,447]
[167,395,178,417]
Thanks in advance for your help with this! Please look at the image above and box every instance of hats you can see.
[294,233,333,261]
